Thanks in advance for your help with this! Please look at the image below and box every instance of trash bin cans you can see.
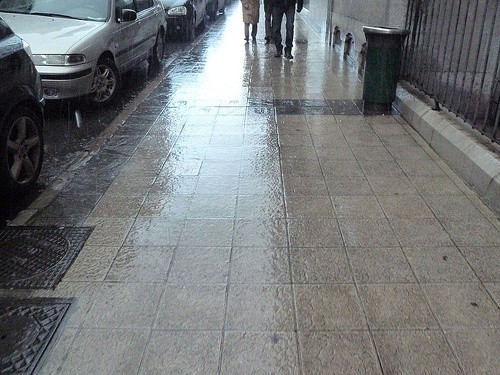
[362,25,411,115]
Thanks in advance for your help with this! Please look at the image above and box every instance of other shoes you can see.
[252,35,256,40]
[265,36,275,43]
[284,52,293,59]
[275,44,283,57]
[244,37,249,41]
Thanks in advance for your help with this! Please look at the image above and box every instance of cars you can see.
[0,0,167,107]
[206,0,228,22]
[0,16,47,190]
[160,0,207,40]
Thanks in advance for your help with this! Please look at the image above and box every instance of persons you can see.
[241,0,260,40]
[264,0,303,59]
